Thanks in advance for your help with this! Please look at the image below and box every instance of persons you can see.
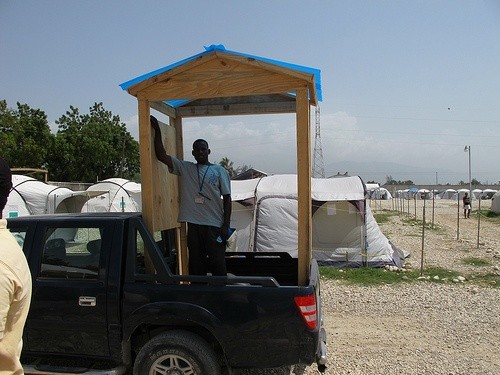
[148,114,236,282]
[0,162,33,375]
[463,192,472,218]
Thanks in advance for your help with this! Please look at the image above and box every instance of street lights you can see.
[465,146,472,204]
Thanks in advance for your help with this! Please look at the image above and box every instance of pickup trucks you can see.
[1,211,328,375]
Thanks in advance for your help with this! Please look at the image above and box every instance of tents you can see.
[364,182,497,199]
[486,189,500,216]
[228,173,410,267]
[2,173,109,245]
[82,176,141,213]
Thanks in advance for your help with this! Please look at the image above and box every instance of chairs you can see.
[44,238,101,269]
